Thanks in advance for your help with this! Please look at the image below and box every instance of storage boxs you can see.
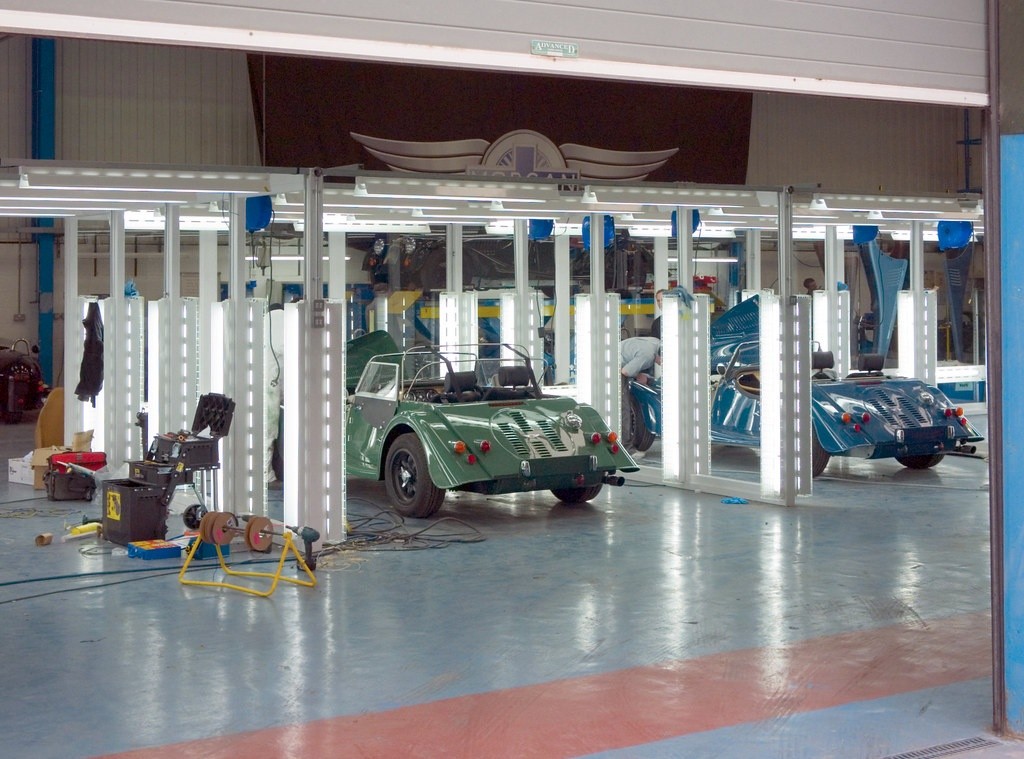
[32,445,92,490]
[7,458,35,485]
[100,392,236,545]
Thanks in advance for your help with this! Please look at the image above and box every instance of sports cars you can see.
[271,327,640,520]
[628,292,986,479]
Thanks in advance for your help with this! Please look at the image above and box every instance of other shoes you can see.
[631,450,646,459]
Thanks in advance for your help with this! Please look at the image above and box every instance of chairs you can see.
[845,355,886,377]
[494,366,533,399]
[444,371,477,394]
[812,350,834,379]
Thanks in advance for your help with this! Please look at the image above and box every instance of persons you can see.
[650,289,668,339]
[803,278,818,296]
[621,337,663,458]
